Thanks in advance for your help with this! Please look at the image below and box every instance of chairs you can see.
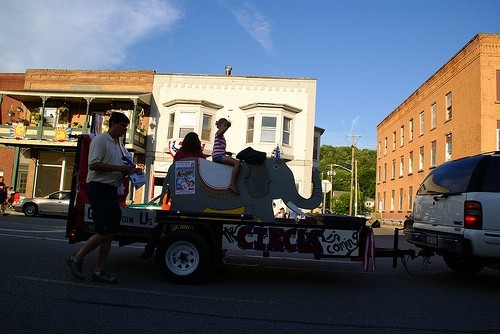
[7,192,21,211]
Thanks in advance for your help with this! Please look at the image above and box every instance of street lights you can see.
[327,163,336,212]
[334,163,353,216]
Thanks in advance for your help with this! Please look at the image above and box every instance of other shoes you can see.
[3,213,8,217]
[229,184,240,195]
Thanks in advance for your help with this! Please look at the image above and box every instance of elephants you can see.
[159,147,322,223]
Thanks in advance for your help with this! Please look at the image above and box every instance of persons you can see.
[67,112,142,284]
[212,118,241,195]
[272,202,305,219]
[0,175,13,216]
[173,132,206,163]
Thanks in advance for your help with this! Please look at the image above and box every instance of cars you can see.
[15,190,71,217]
[404,212,415,228]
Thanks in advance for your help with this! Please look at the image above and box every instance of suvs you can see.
[404,152,500,271]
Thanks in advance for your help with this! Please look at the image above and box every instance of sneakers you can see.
[66,254,86,282]
[90,270,117,284]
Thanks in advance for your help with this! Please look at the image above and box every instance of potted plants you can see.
[17,106,24,112]
[71,122,78,126]
[8,111,14,116]
[138,113,144,121]
[31,112,35,120]
